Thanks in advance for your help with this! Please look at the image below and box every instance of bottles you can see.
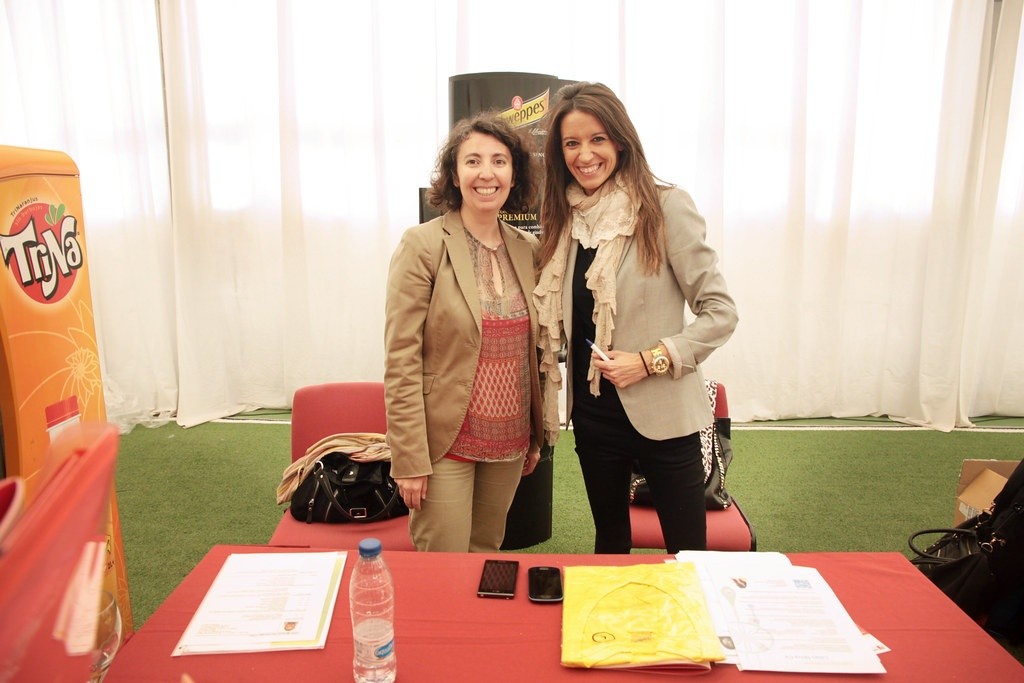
[45,394,118,644]
[348,538,397,683]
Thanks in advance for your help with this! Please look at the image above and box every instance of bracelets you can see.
[639,351,650,377]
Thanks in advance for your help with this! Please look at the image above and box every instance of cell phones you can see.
[527,566,564,603]
[477,560,519,600]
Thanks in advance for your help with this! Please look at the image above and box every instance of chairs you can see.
[272,382,454,548]
[633,380,758,550]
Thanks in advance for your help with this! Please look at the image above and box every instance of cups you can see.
[83,589,123,683]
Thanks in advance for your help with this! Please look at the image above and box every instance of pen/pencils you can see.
[584,336,611,361]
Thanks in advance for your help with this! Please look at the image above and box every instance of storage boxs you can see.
[957,459,1022,530]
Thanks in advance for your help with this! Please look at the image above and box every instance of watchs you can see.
[649,348,670,376]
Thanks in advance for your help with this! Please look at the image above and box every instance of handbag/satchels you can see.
[630,417,733,510]
[908,457,1024,646]
[291,453,409,523]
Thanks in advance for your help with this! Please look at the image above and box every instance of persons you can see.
[382,111,541,554]
[529,81,738,559]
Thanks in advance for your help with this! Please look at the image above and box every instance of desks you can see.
[103,545,1023,682]
